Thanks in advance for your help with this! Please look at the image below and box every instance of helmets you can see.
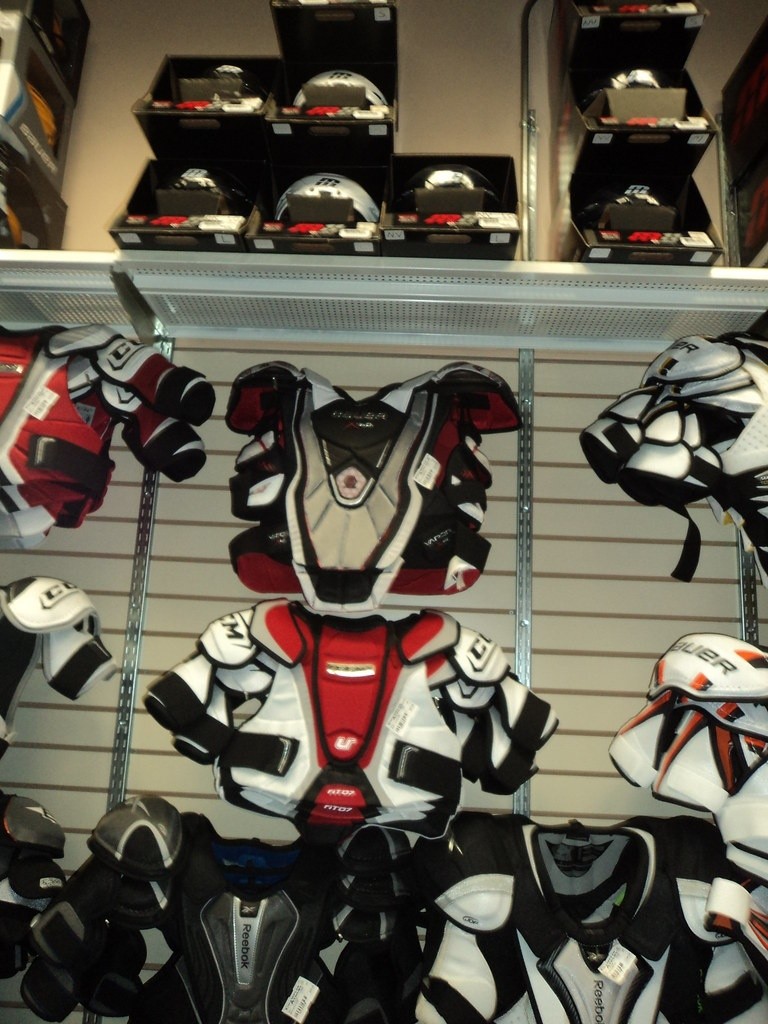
[170,168,261,219]
[24,81,58,147]
[605,183,662,208]
[275,172,381,223]
[401,163,497,213]
[204,64,269,101]
[0,201,23,250]
[610,68,660,89]
[292,69,387,109]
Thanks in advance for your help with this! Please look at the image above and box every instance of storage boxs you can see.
[0,0,767,268]
